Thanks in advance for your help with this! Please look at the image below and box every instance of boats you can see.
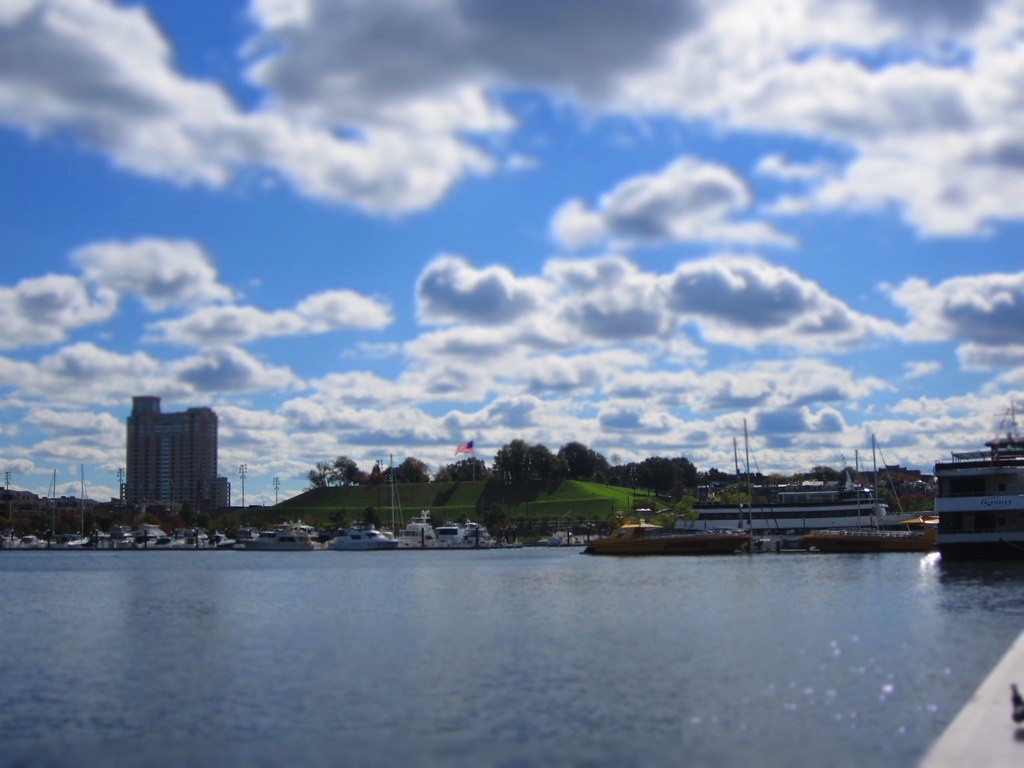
[580,518,751,559]
[932,398,1024,566]
[676,418,889,550]
[549,536,561,546]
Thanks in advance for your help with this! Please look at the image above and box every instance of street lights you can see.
[630,465,637,502]
[116,467,124,508]
[4,470,12,495]
[272,475,281,506]
[239,464,248,507]
[168,480,175,517]
[502,467,512,517]
[384,473,392,505]
[376,459,384,519]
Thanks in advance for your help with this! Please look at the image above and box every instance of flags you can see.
[454,441,473,456]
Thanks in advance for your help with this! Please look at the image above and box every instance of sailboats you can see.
[0,461,526,551]
[801,433,937,552]
